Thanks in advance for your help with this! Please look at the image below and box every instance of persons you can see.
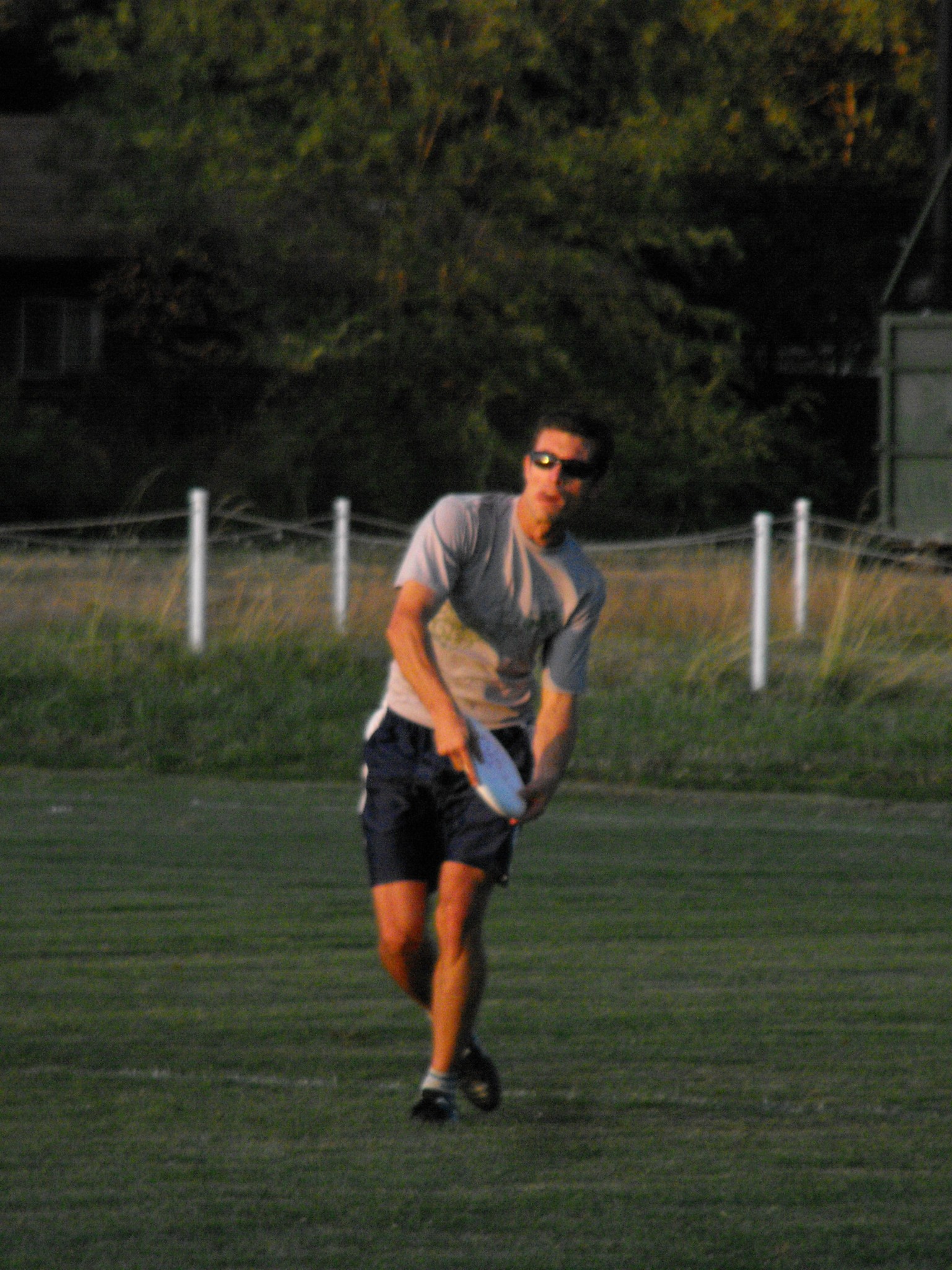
[362,410,606,1121]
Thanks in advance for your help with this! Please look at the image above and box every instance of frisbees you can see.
[458,709,531,820]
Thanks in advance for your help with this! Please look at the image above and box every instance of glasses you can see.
[530,449,595,479]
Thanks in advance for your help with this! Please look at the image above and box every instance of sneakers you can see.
[410,1087,451,1122]
[451,1037,500,1110]
